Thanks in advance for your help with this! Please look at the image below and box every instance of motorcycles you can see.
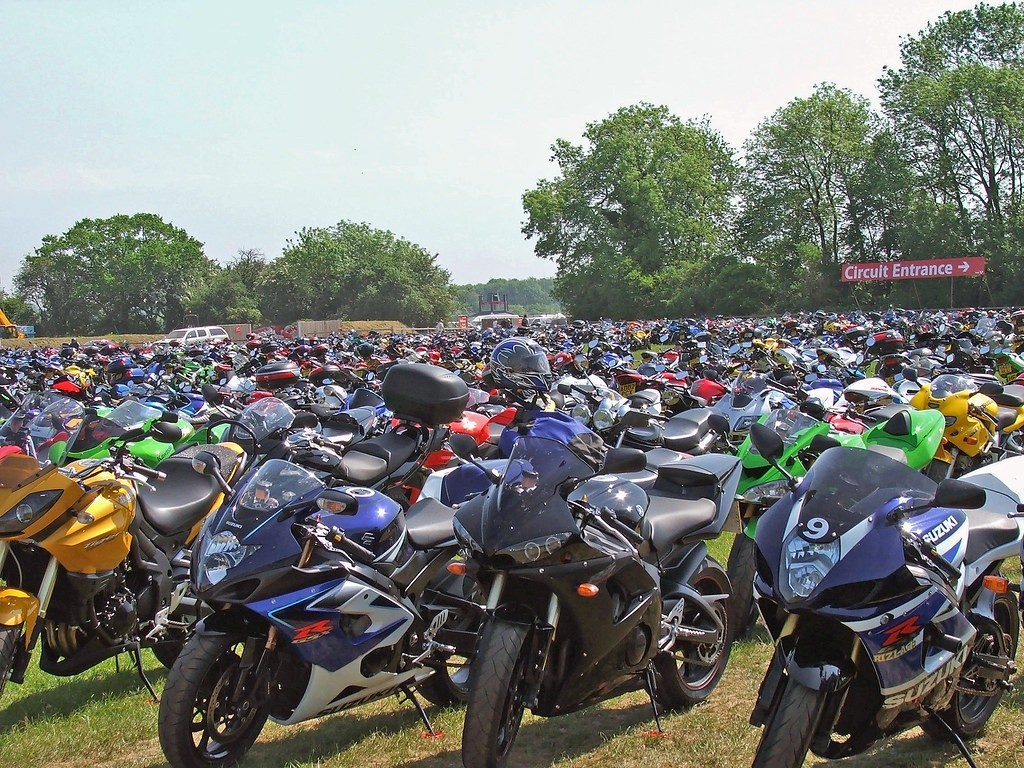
[157,452,538,768]
[447,435,744,768]
[1,391,257,702]
[748,421,1024,768]
[0,307,1024,646]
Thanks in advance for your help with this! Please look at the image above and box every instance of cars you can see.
[146,326,230,346]
[79,340,120,351]
[533,317,567,327]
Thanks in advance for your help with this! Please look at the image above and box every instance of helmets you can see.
[490,337,553,391]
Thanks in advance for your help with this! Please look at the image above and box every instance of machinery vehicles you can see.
[0,309,26,339]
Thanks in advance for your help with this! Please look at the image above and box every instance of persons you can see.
[436,319,445,337]
[70,338,79,351]
[521,315,529,327]
[492,318,510,329]
